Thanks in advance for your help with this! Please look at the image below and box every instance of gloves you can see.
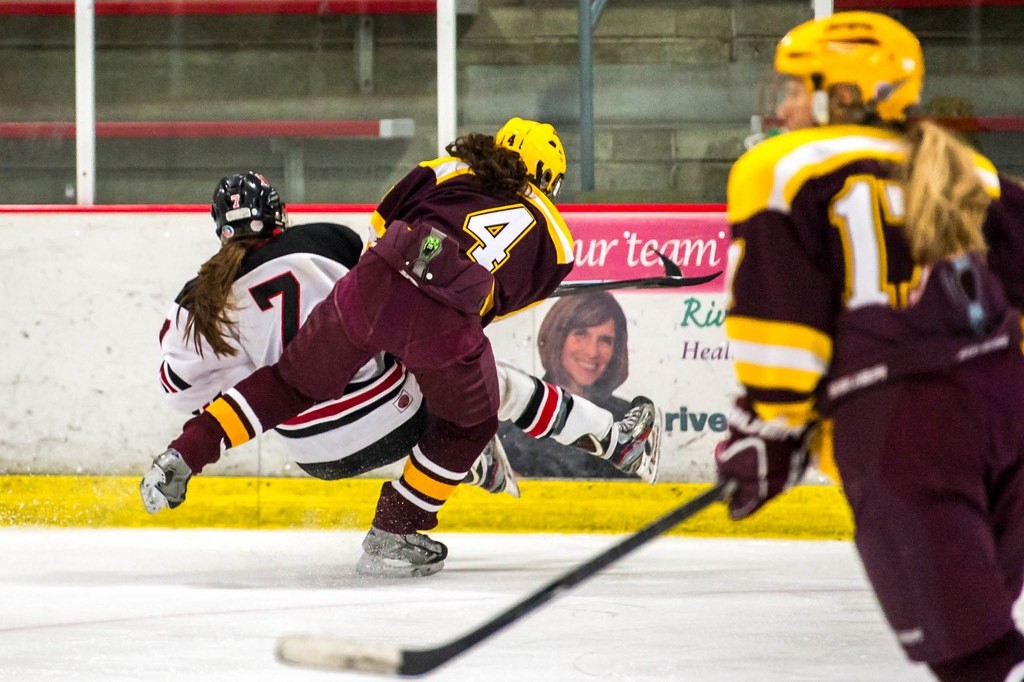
[713,395,824,520]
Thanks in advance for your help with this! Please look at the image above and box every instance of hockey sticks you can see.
[277,478,734,681]
[552,246,724,297]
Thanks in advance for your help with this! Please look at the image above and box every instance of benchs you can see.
[753,114,1023,131]
[811,0,1024,12]
[0,0,481,96]
[0,120,413,203]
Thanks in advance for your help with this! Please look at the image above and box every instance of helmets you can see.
[494,116,567,205]
[771,9,925,128]
[210,168,285,245]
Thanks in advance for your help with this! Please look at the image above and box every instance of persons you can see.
[494,290,642,480]
[715,10,1024,682]
[156,169,660,498]
[140,117,576,579]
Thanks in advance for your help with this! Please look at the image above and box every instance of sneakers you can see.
[353,522,449,577]
[138,446,194,514]
[565,396,665,486]
[467,431,522,499]
